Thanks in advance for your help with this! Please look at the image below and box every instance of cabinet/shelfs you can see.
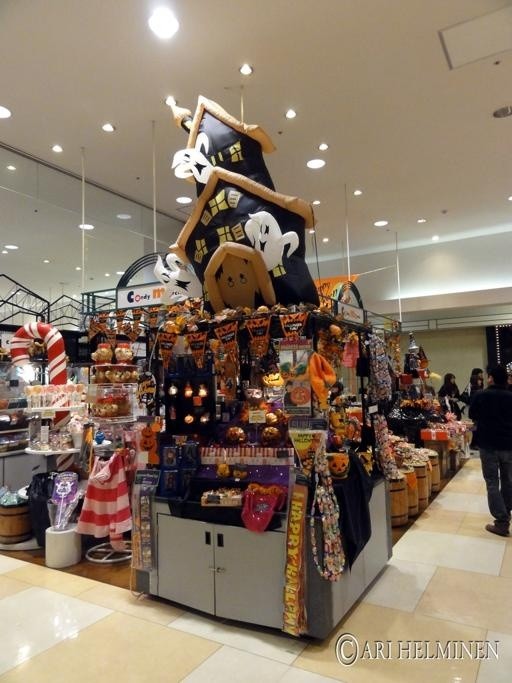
[1,296,400,643]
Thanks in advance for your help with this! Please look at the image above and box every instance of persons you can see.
[461,364,489,450]
[469,363,511,536]
[437,371,461,419]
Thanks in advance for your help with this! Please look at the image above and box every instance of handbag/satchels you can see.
[400,353,431,397]
[460,392,470,403]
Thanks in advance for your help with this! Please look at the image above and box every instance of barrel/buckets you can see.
[0,499,33,545]
[428,454,441,493]
[410,462,428,509]
[389,472,408,526]
[404,467,420,516]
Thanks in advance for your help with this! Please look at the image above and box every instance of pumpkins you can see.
[225,426,246,444]
[262,427,282,446]
[329,454,348,473]
[261,372,285,388]
[139,426,159,464]
[290,385,311,405]
[266,413,279,424]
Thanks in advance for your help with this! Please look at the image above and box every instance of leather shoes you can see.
[487,524,510,535]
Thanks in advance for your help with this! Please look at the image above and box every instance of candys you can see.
[23,383,84,408]
